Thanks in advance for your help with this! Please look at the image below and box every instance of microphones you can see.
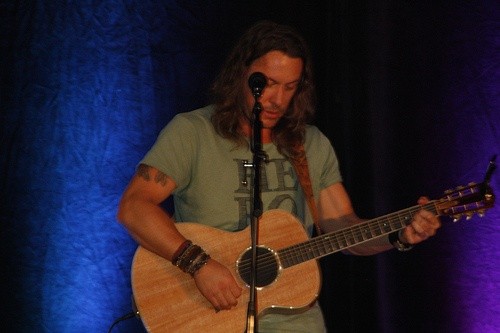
[248,72,267,113]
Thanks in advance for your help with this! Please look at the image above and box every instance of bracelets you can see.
[389,232,413,251]
[171,241,209,276]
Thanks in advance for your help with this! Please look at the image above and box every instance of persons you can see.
[117,26,440,333]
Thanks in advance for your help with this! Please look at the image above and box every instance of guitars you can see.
[129,154,497,333]
